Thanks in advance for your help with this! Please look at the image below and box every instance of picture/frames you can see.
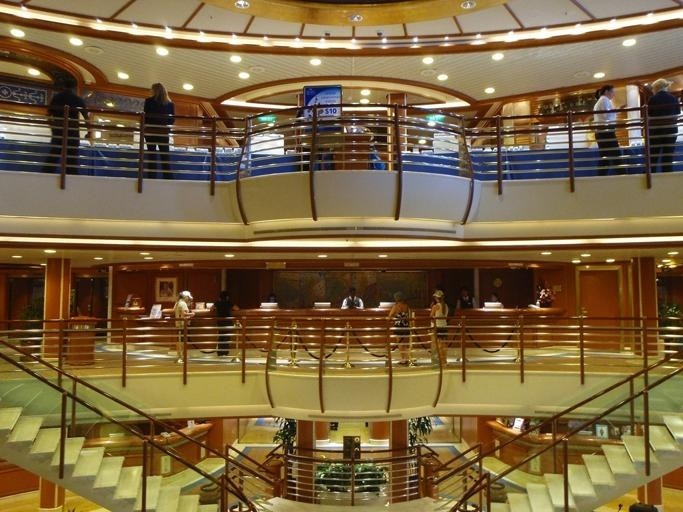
[154,274,178,303]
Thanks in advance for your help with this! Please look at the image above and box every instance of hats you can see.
[180,290,194,302]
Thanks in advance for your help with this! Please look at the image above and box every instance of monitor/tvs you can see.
[206,303,214,309]
[380,302,396,307]
[314,302,331,307]
[109,433,124,437]
[578,431,592,436]
[484,302,502,307]
[261,303,278,309]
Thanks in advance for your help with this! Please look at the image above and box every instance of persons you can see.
[592,85,629,176]
[209,290,241,357]
[266,292,278,303]
[174,290,195,364]
[141,83,177,178]
[455,286,478,309]
[428,289,449,368]
[489,291,503,305]
[599,425,605,437]
[646,78,681,172]
[294,107,332,170]
[41,77,96,175]
[161,281,174,298]
[340,286,365,310]
[387,290,412,365]
